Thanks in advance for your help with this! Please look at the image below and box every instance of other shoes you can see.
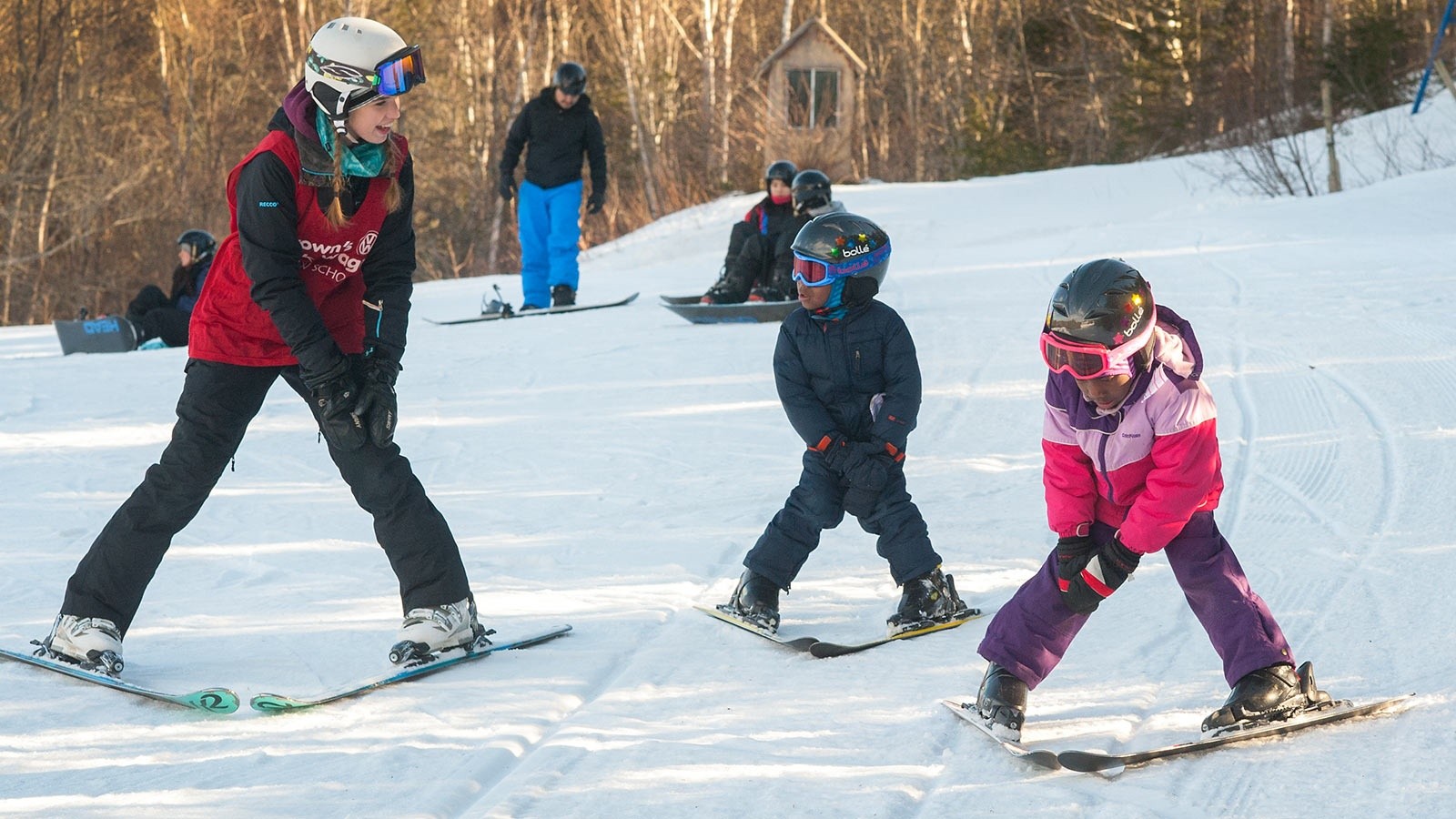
[518,305,539,310]
[551,285,576,306]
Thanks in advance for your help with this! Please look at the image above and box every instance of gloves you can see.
[499,174,518,201]
[825,435,888,491]
[1057,535,1100,614]
[843,435,906,518]
[304,364,365,452]
[586,191,604,214]
[1066,539,1141,611]
[355,345,405,449]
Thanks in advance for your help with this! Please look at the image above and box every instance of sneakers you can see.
[727,569,780,631]
[708,270,730,292]
[388,596,474,664]
[896,567,949,624]
[1202,664,1308,733]
[700,285,743,304]
[50,613,124,675]
[977,661,1028,730]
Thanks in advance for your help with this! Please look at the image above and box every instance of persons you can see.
[496,63,607,312]
[45,19,485,678]
[699,161,847,306]
[976,258,1302,746]
[78,228,218,347]
[876,257,880,262]
[727,211,958,636]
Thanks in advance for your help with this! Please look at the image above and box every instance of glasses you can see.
[791,251,835,287]
[1040,330,1112,381]
[372,44,426,96]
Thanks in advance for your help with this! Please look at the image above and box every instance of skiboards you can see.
[0,623,573,720]
[935,691,1407,771]
[700,605,1006,658]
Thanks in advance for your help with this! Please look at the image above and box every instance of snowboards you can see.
[660,294,759,304]
[419,290,641,324]
[664,300,805,324]
[53,317,136,354]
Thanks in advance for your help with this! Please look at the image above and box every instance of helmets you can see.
[764,162,798,192]
[1045,260,1154,348]
[175,229,217,262]
[553,63,587,96]
[790,212,891,293]
[303,17,406,119]
[790,168,833,211]
